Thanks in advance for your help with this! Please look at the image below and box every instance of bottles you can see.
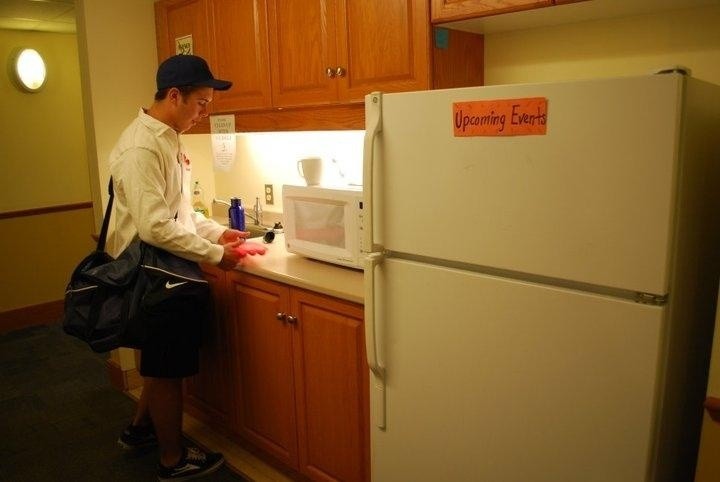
[193,181,209,216]
[228,196,246,230]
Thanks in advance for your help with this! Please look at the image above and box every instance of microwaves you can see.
[279,182,369,270]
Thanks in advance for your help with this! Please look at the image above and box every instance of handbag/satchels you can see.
[61,237,213,352]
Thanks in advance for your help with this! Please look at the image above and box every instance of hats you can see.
[156,54,233,91]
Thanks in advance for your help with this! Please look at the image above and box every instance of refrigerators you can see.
[356,70,719,481]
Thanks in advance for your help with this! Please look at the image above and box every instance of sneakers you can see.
[117,423,156,449]
[155,446,225,482]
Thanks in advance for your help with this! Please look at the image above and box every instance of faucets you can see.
[213,197,262,224]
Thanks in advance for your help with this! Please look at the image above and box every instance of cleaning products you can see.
[191,180,209,217]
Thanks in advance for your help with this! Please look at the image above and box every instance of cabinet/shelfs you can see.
[183,259,370,482]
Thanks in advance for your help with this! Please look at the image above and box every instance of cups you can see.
[295,157,323,186]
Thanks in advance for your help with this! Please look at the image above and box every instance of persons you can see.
[108,54,250,481]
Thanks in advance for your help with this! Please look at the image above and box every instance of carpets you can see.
[1,320,254,482]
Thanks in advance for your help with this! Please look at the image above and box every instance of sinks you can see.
[224,222,269,241]
